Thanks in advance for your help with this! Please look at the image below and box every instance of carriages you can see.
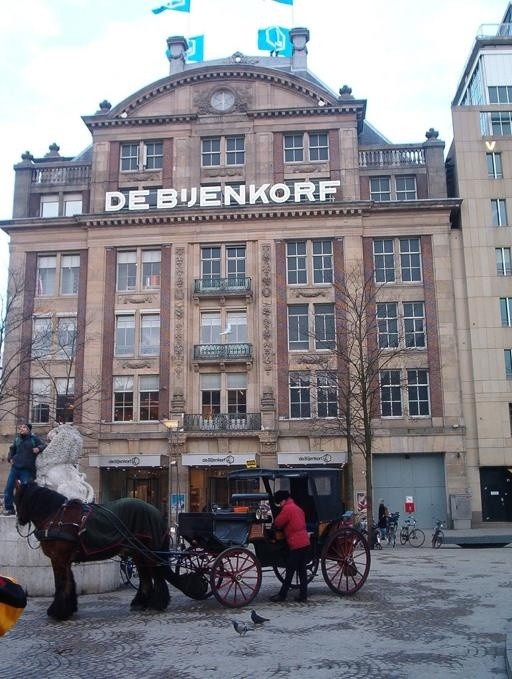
[13,467,371,619]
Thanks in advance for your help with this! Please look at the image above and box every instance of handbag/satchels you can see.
[385,508,389,516]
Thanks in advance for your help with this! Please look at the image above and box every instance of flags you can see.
[274,0,294,6]
[166,35,203,62]
[257,25,292,58]
[151,0,190,15]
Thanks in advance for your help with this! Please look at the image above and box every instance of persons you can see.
[2,422,48,516]
[270,489,315,603]
[377,497,391,542]
[356,492,367,511]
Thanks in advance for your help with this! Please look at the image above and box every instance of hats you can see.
[378,498,385,505]
[24,422,32,432]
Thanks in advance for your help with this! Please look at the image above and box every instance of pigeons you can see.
[250,609,270,626]
[231,620,254,637]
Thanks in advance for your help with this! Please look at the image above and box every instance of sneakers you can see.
[269,593,286,602]
[294,596,308,603]
[380,537,385,540]
[2,509,16,516]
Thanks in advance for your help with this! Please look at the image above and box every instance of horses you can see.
[12,478,170,623]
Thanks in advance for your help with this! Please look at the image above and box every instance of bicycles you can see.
[383,514,446,549]
